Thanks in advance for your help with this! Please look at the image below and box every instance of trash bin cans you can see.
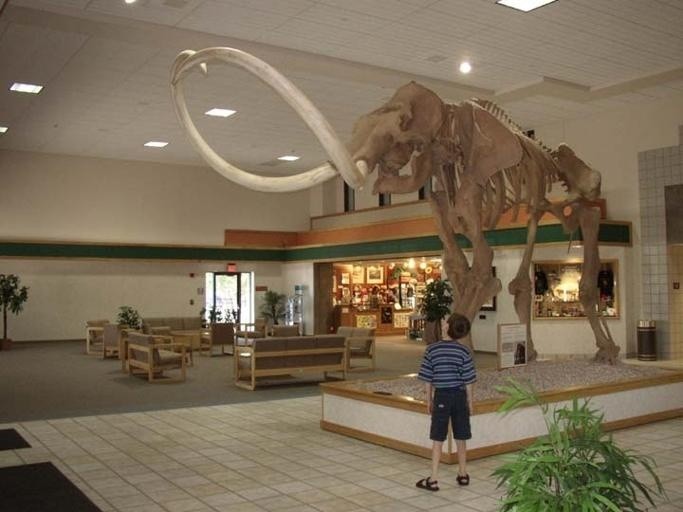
[636,320,658,361]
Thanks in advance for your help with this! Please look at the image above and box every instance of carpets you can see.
[1,428,31,450]
[0,462,102,512]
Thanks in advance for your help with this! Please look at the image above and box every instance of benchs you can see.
[233,334,347,390]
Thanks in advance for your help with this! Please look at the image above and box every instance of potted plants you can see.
[0,273,30,351]
[257,290,288,336]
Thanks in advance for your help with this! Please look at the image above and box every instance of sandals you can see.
[416,477,440,491]
[456,472,471,485]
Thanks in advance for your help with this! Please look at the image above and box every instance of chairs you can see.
[271,324,298,337]
[85,316,236,391]
[330,325,376,372]
[236,317,267,338]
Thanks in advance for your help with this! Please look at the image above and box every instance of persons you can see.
[414,313,478,491]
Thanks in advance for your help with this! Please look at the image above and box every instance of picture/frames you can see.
[366,263,384,284]
